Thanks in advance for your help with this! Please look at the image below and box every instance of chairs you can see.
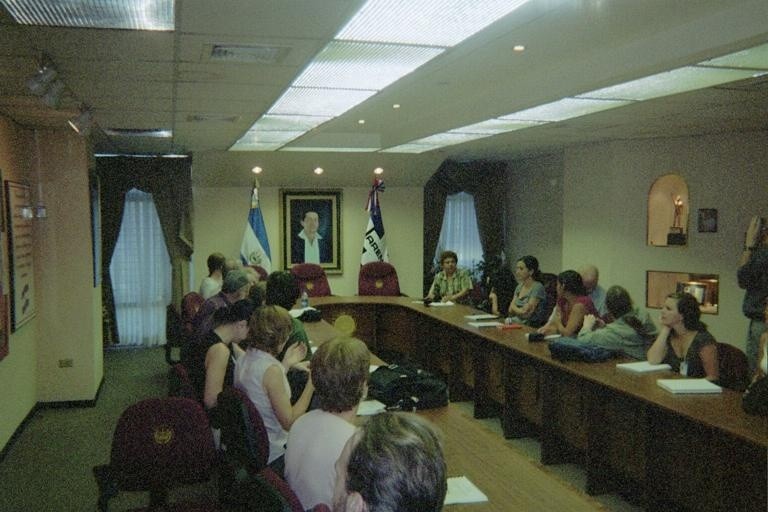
[92,397,223,511]
[255,467,309,512]
[719,343,748,388]
[180,292,206,324]
[166,301,181,364]
[165,363,194,397]
[215,388,270,467]
[254,265,267,281]
[292,263,333,297]
[360,261,401,296]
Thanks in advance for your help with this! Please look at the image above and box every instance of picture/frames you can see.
[278,188,343,276]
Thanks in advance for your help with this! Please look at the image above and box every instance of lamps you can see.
[67,105,97,135]
[26,61,59,97]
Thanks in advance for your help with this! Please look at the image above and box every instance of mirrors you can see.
[647,173,690,247]
[646,271,719,314]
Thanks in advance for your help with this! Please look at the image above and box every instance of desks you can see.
[399,297,766,511]
[295,296,400,369]
[298,312,611,512]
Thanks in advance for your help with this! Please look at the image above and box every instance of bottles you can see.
[301,292,309,308]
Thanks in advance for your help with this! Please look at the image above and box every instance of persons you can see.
[284,337,370,511]
[736,215,768,418]
[421,251,474,308]
[331,411,448,511]
[463,256,660,362]
[671,190,684,227]
[647,292,721,383]
[290,208,331,264]
[616,361,672,373]
[181,253,317,484]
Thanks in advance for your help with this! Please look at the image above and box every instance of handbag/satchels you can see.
[547,336,617,362]
[368,365,449,409]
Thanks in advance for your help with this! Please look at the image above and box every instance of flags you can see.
[240,178,273,282]
[360,179,389,268]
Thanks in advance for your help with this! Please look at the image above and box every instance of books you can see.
[655,378,723,394]
[355,399,388,417]
[443,475,489,506]
